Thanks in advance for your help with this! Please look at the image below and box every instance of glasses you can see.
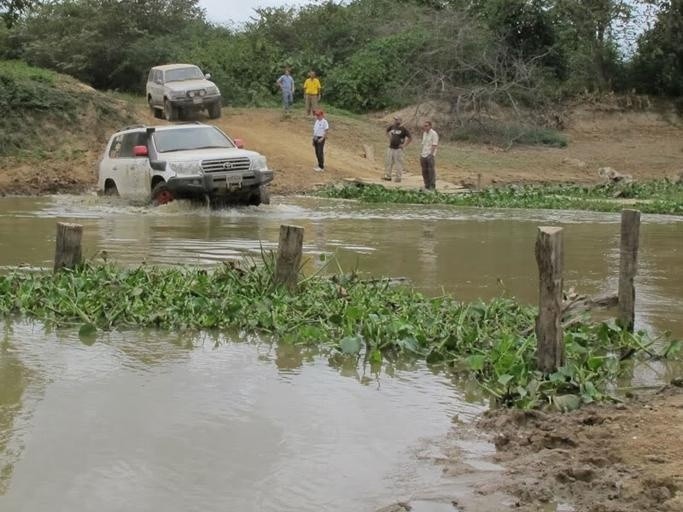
[424,125,430,127]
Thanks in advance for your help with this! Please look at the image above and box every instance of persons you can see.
[303,72,322,117]
[420,121,439,190]
[312,109,330,171]
[276,68,295,115]
[381,115,412,182]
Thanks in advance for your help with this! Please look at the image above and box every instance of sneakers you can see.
[313,166,324,172]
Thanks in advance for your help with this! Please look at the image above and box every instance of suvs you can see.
[143,62,223,122]
[92,122,276,209]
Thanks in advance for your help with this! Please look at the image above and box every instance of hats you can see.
[315,110,323,116]
[393,115,403,121]
